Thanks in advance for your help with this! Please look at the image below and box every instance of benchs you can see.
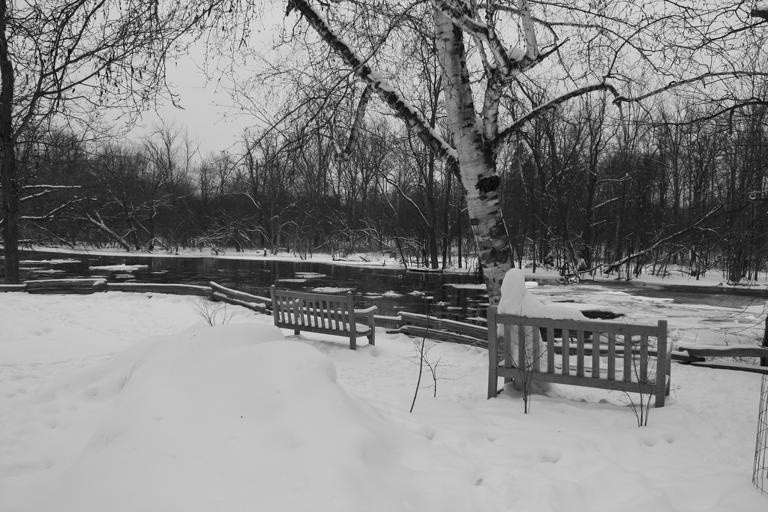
[270,284,378,350]
[485,307,673,408]
